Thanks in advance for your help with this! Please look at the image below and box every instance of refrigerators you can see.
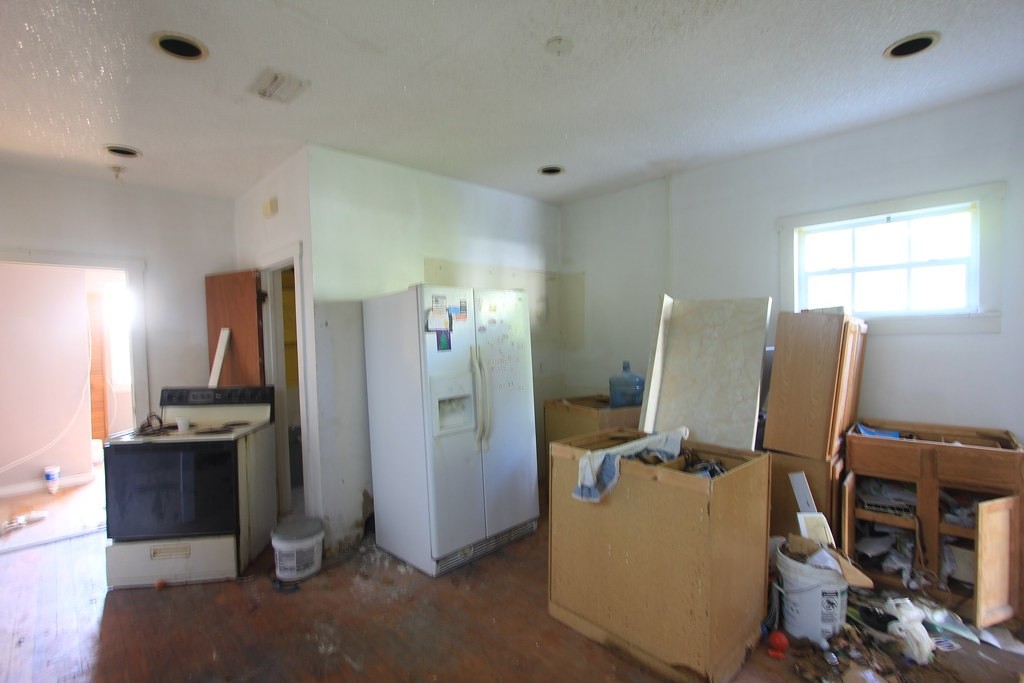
[362,283,540,578]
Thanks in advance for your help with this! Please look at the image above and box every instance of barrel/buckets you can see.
[770,539,848,641]
[45,465,61,494]
[270,517,326,582]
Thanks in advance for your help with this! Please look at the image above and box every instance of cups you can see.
[176,417,189,432]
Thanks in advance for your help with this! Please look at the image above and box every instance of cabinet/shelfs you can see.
[542,294,1024,683]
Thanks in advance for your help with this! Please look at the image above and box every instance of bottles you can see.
[610,361,645,408]
